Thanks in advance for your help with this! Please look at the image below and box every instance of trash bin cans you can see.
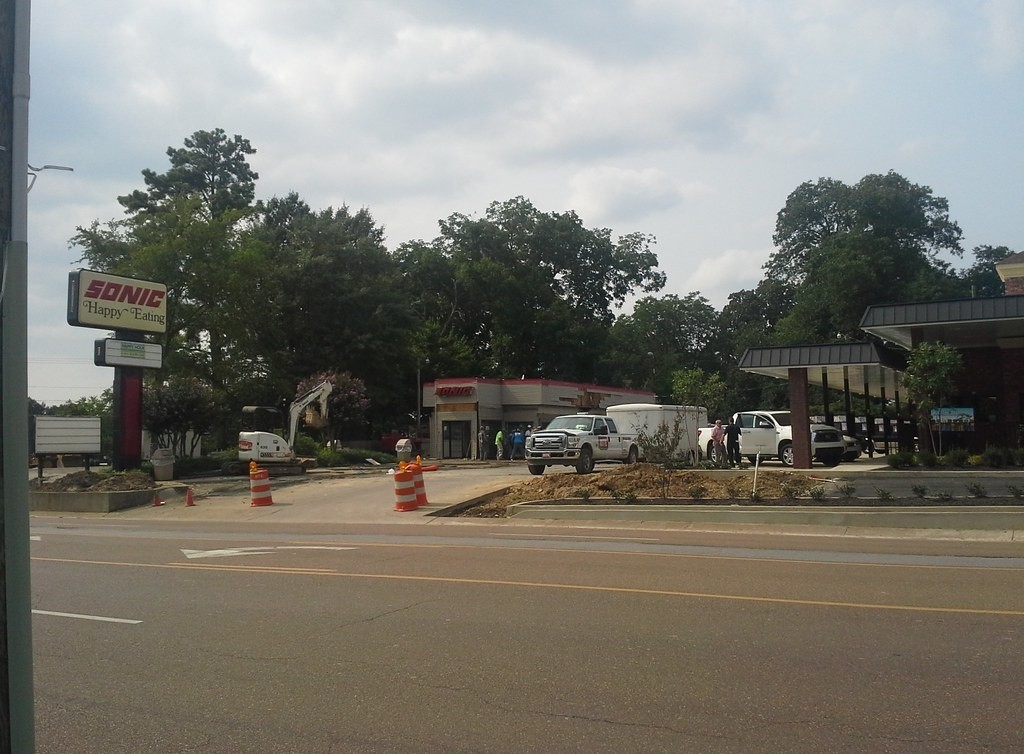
[150,446,175,481]
[395,439,412,462]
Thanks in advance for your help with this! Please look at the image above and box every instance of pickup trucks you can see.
[698,410,846,467]
[524,412,641,476]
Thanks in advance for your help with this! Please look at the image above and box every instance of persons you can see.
[479,424,542,460]
[720,418,741,462]
[711,420,728,462]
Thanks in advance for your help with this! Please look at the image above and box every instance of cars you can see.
[843,436,861,462]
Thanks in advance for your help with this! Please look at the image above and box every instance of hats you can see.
[527,425,531,427]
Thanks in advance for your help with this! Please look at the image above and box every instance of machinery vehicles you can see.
[239,378,346,466]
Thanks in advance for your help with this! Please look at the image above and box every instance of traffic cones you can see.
[153,493,165,506]
[185,489,196,508]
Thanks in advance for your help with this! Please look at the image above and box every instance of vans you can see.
[607,404,706,468]
